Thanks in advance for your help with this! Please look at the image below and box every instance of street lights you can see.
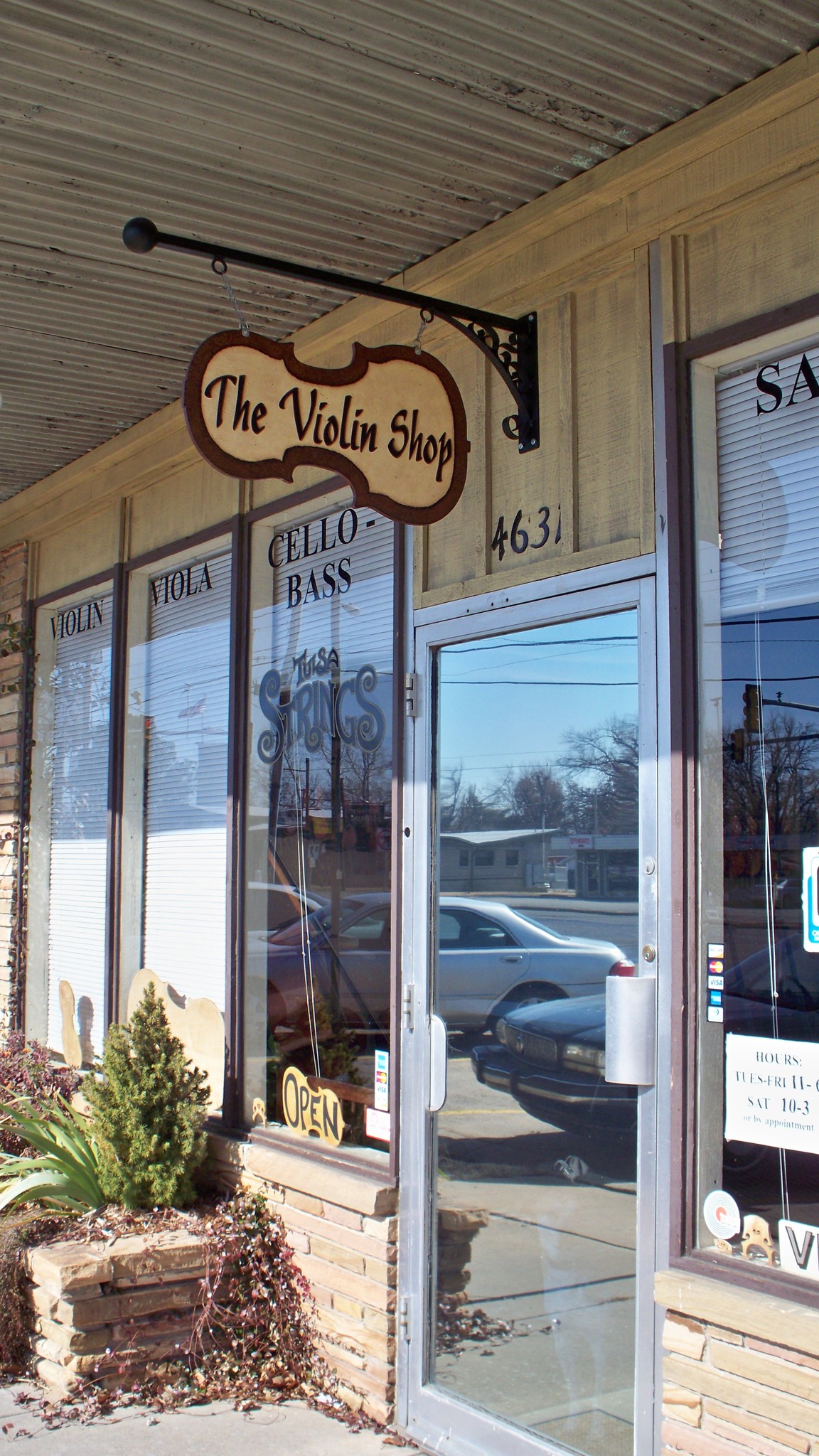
[329,593,362,1035]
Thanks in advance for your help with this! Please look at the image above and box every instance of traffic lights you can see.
[143,718,151,743]
[730,728,745,764]
[742,684,765,733]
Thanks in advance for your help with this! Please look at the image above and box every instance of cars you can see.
[245,888,636,1039]
[547,854,640,890]
[470,930,819,1190]
[745,876,803,910]
[244,880,385,940]
